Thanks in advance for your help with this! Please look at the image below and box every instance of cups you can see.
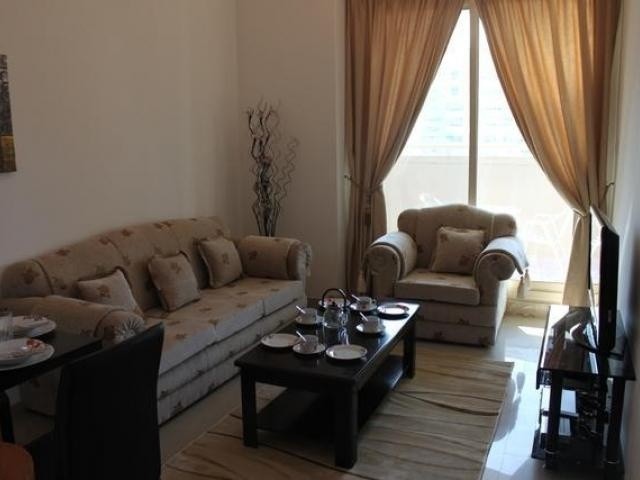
[362,316,380,333]
[301,335,319,353]
[300,307,317,323]
[357,297,376,310]
[0,311,15,342]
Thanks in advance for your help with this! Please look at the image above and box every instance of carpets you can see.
[159,345,514,480]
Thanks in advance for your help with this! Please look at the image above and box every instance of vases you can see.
[252,200,280,237]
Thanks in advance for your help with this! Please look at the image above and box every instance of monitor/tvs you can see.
[585,205,621,353]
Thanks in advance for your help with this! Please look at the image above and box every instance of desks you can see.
[537,304,636,480]
[0,308,103,445]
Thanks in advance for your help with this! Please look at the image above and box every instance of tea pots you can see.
[322,287,349,328]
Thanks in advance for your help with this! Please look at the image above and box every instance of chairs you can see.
[362,203,529,346]
[22,321,165,480]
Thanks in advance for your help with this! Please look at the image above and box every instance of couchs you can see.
[0,215,313,428]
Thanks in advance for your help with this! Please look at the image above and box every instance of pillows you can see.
[428,225,487,274]
[77,266,144,318]
[148,249,201,312]
[198,235,243,288]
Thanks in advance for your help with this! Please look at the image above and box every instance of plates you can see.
[318,298,351,309]
[377,303,409,315]
[261,333,299,348]
[356,323,386,334]
[0,316,57,338]
[350,302,377,312]
[0,343,55,371]
[292,343,325,354]
[325,344,368,360]
[295,315,323,324]
